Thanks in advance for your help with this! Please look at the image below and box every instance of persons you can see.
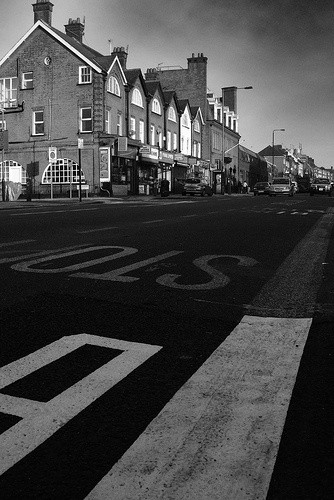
[238,180,249,194]
[148,175,158,197]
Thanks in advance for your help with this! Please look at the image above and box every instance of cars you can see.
[267,177,295,197]
[253,182,272,197]
[310,177,334,197]
[182,177,214,197]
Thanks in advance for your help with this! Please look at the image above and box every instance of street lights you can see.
[271,127,287,179]
[221,86,254,195]
